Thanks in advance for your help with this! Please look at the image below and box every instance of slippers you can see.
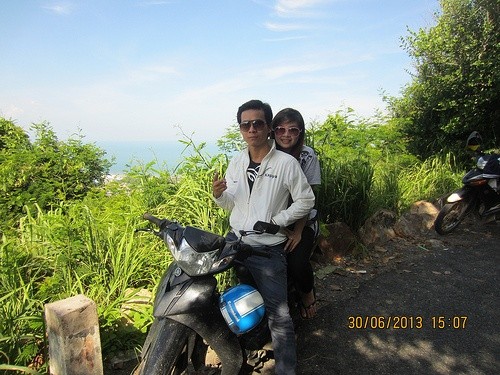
[300,286,316,319]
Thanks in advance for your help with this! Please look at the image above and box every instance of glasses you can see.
[272,127,302,137]
[240,120,267,132]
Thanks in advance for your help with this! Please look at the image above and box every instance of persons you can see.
[211,100,315,375]
[270,108,321,320]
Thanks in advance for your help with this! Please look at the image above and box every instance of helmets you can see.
[219,284,265,333]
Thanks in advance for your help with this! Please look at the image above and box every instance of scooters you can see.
[130,208,322,375]
[433,146,500,236]
